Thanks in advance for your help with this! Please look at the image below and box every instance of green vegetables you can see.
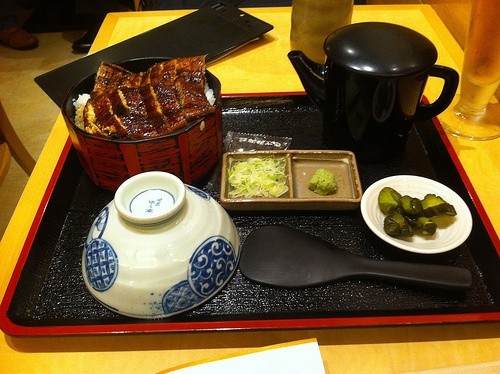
[227,156,289,198]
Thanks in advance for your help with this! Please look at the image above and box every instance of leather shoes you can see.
[0,24,39,49]
[72,26,102,52]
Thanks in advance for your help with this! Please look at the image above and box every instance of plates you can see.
[359,175,473,253]
[220,149,363,210]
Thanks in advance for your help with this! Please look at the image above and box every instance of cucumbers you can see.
[378,187,458,237]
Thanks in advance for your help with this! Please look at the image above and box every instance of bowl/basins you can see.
[60,56,223,193]
[81,171,242,321]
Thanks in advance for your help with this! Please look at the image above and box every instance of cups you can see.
[437,0,500,141]
[290,0,354,65]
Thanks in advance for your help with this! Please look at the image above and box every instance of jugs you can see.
[286,23,459,165]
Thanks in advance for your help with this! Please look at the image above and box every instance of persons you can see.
[0,0,136,53]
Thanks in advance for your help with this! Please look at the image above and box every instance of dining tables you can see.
[0,3,500,374]
